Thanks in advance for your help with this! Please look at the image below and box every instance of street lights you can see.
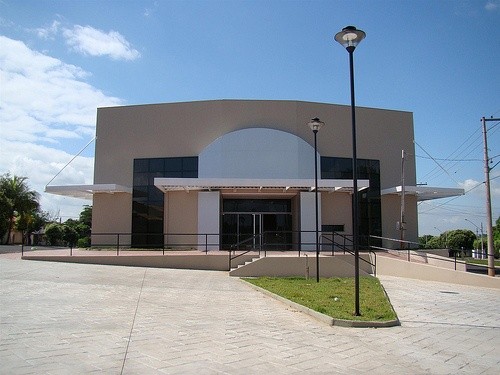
[465,218,485,260]
[333,26,367,316]
[308,117,325,283]
